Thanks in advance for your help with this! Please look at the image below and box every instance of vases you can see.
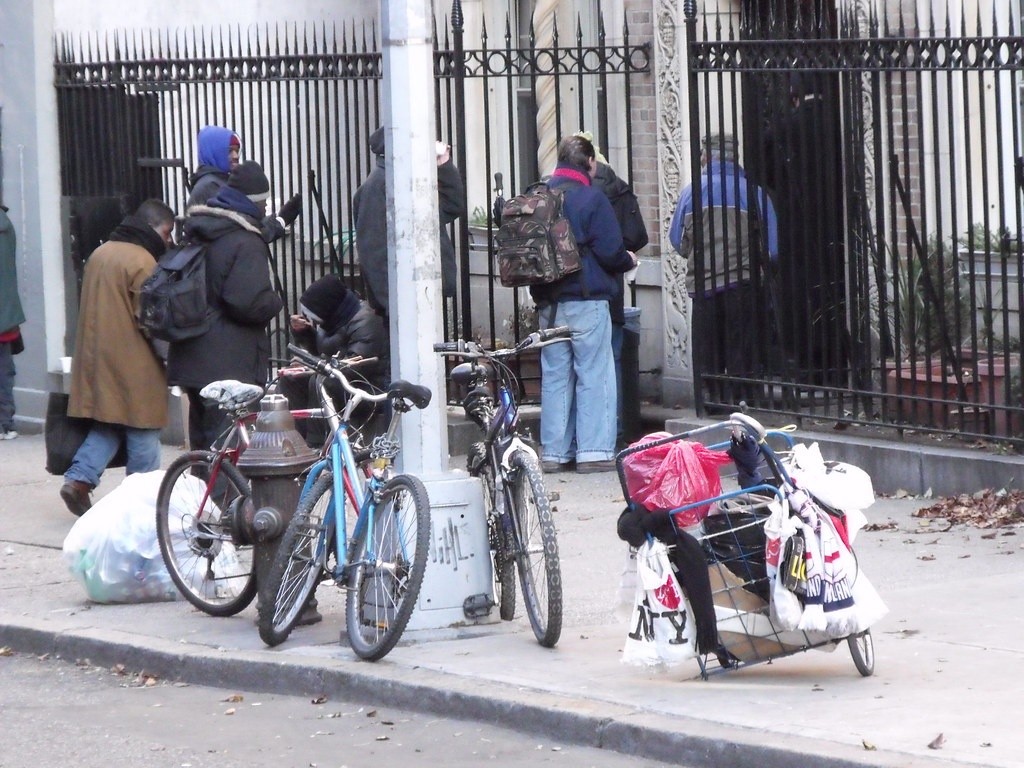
[887,366,981,431]
[949,406,989,439]
[978,357,1024,441]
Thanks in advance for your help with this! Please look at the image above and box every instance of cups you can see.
[171,386,183,397]
[625,261,642,281]
[436,142,446,155]
[62,357,72,373]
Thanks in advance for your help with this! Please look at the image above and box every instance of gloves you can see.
[278,193,301,226]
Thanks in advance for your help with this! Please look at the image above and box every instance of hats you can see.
[227,160,269,202]
[230,134,241,148]
[369,125,385,154]
[699,133,739,157]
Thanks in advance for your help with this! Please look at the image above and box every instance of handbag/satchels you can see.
[622,432,734,528]
[765,442,887,636]
[623,539,696,666]
[45,392,128,476]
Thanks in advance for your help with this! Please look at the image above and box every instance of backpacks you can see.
[495,182,589,287]
[140,236,221,342]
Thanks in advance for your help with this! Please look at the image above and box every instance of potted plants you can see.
[871,235,975,376]
[468,206,500,251]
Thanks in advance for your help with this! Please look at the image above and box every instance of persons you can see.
[352,125,465,326]
[0,206,25,440]
[762,73,848,389]
[669,131,779,415]
[529,132,648,475]
[60,199,175,516]
[274,273,391,449]
[167,124,302,512]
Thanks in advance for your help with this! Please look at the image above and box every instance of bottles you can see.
[135,570,178,601]
[80,548,105,601]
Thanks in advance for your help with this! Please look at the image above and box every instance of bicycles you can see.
[258,347,431,663]
[434,329,572,648]
[156,348,381,615]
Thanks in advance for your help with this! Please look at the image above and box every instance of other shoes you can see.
[60,479,91,516]
[541,458,617,473]
[0,431,17,440]
